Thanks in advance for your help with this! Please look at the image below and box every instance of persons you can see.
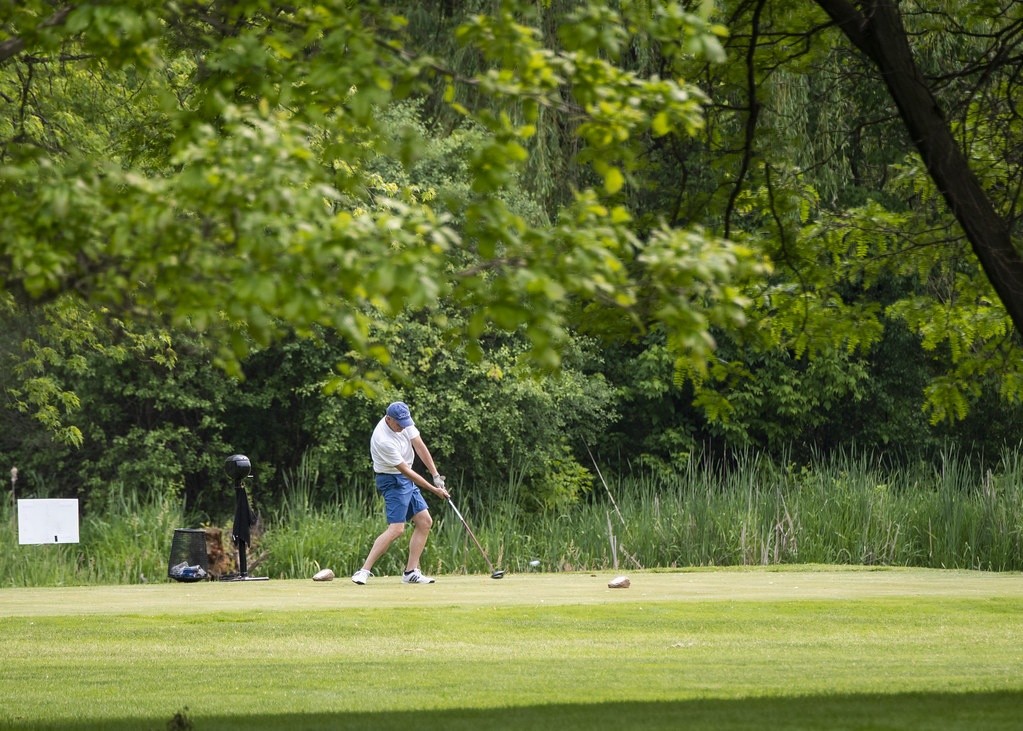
[350,401,450,586]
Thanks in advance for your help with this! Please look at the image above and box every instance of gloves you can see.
[432,474,446,489]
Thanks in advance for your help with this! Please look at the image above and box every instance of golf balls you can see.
[529,560,541,567]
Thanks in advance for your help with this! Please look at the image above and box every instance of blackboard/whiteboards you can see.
[18,498,79,545]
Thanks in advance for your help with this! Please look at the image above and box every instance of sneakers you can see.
[402,568,435,583]
[352,569,374,585]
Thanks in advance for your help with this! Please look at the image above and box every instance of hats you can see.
[387,402,414,427]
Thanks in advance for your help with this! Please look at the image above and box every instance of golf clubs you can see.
[439,486,505,579]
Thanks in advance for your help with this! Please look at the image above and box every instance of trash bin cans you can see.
[168,527,208,583]
